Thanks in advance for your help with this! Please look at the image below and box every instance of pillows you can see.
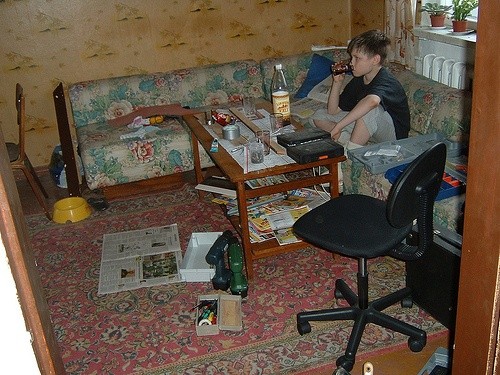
[293,52,353,98]
[308,73,353,103]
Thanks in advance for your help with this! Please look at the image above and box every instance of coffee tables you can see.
[183,102,347,280]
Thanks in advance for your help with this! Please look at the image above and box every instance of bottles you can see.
[230,272,249,297]
[229,235,244,273]
[205,230,231,264]
[211,252,231,291]
[271,63,292,128]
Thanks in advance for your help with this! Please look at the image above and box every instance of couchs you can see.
[260,48,471,242]
[54,58,266,207]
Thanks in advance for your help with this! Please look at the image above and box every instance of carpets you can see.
[24,171,449,374]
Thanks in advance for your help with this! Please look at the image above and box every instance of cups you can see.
[330,58,355,75]
[269,113,285,139]
[243,96,257,119]
[204,108,214,125]
[254,131,270,155]
[221,125,240,140]
[248,138,264,164]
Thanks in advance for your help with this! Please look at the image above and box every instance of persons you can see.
[312,29,410,196]
[121,269,135,278]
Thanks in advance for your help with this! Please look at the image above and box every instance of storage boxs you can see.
[179,231,222,282]
[195,294,242,336]
[346,133,465,175]
[418,346,451,375]
[383,161,466,202]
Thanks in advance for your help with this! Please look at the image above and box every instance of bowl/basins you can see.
[51,197,92,223]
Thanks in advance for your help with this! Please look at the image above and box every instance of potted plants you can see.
[417,2,452,27]
[450,0,479,32]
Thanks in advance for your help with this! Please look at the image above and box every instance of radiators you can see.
[414,52,475,91]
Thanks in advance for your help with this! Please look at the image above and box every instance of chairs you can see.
[292,143,446,375]
[5,83,52,221]
